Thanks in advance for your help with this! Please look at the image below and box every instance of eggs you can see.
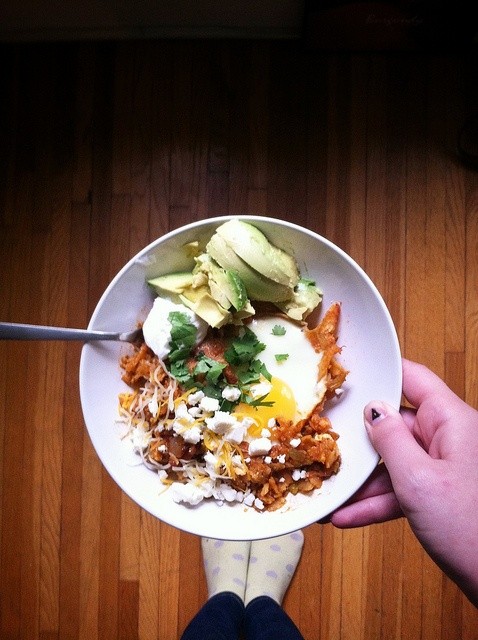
[224,315,329,448]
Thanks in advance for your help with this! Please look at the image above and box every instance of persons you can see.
[177,356,478,640]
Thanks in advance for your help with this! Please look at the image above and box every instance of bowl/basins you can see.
[78,215,403,540]
